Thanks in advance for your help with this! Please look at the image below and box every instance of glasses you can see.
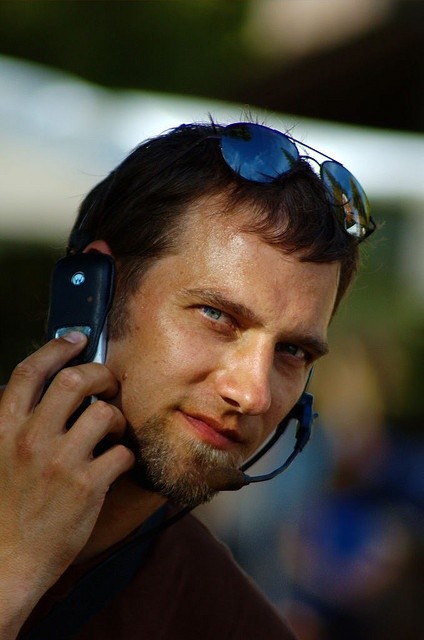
[95,123,376,247]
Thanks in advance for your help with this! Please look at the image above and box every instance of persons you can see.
[1,102,386,640]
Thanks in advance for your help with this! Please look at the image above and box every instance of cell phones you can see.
[41,254,112,456]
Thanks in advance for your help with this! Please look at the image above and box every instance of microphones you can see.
[207,393,318,492]
